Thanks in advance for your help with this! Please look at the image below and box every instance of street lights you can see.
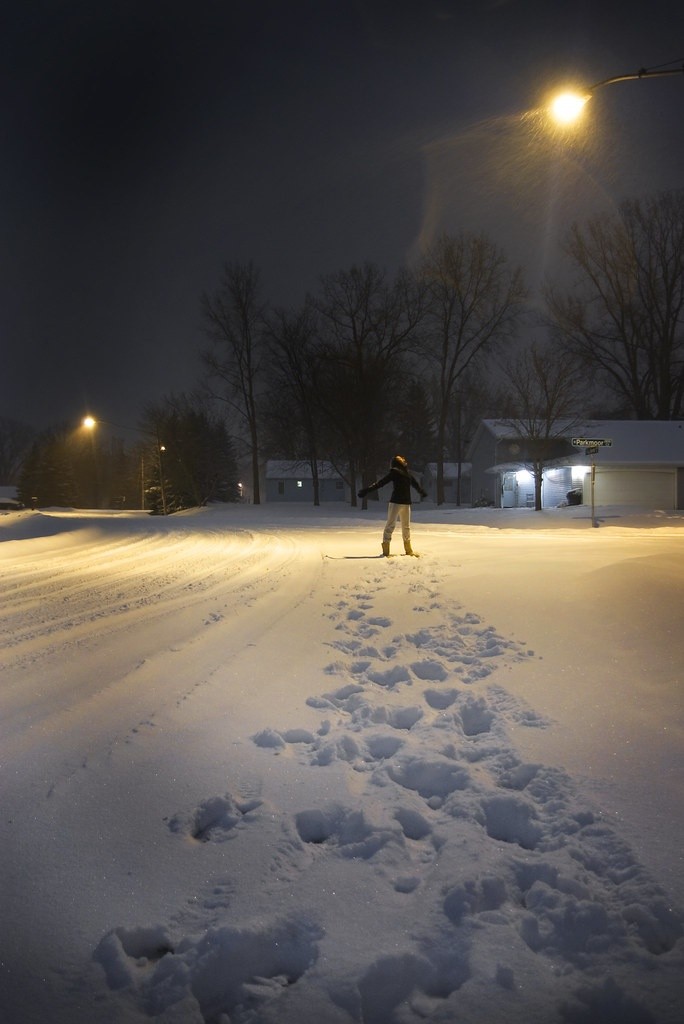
[83,416,168,516]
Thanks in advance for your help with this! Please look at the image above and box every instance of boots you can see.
[381,542,390,556]
[404,540,419,558]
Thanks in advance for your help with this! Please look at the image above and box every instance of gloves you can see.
[358,487,369,498]
[419,489,428,497]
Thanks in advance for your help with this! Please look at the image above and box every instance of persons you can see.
[358,455,429,557]
